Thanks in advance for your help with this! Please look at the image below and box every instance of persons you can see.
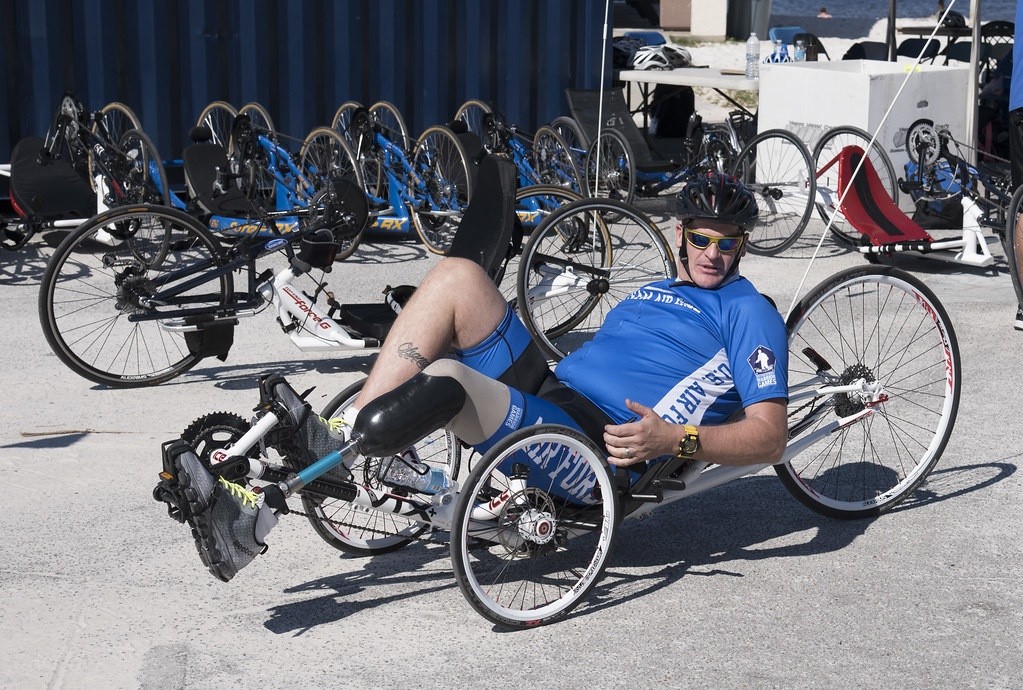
[817,8,832,19]
[262,174,788,518]
[1008,0,1023,330]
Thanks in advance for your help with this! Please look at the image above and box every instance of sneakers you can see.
[271,380,359,503]
[175,449,268,582]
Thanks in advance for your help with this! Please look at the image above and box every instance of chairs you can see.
[770,10,1015,159]
[434,120,481,179]
[5,137,94,218]
[624,30,667,118]
[177,127,272,219]
[562,86,685,172]
[337,159,519,336]
[836,143,933,248]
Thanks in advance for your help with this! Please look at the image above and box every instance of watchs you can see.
[675,424,700,461]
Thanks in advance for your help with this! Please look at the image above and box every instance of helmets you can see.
[938,10,966,28]
[676,174,760,232]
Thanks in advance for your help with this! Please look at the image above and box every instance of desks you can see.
[618,70,758,152]
[898,26,1014,90]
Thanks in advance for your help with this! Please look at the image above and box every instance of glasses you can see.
[684,227,744,252]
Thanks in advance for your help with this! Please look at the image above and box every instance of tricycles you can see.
[0,91,1023,630]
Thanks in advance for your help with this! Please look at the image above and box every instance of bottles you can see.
[794,40,805,62]
[374,454,459,495]
[775,39,782,63]
[781,43,787,62]
[745,32,760,81]
